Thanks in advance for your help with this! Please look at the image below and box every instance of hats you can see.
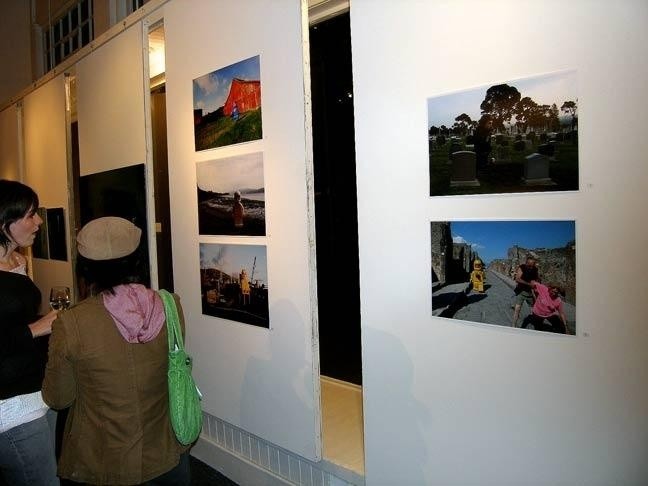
[72,216,143,260]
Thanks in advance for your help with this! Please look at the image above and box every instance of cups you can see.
[49,285,72,312]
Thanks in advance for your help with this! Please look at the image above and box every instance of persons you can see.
[472,113,498,193]
[0,178,69,486]
[37,214,202,486]
[520,279,569,335]
[511,255,540,328]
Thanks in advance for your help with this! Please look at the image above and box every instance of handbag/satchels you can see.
[156,287,204,445]
